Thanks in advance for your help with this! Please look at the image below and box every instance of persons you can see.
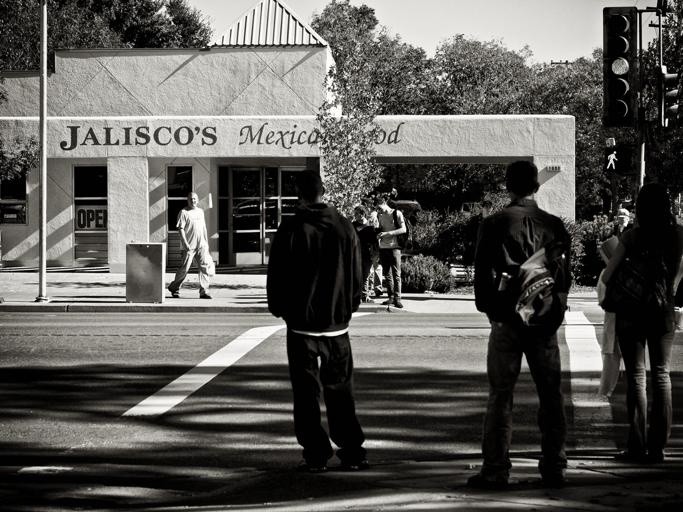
[266,170,368,472]
[376,195,407,308]
[595,220,637,425]
[466,158,572,492]
[602,183,681,466]
[165,190,212,299]
[351,205,379,304]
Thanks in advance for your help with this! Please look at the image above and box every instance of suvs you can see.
[226,197,301,236]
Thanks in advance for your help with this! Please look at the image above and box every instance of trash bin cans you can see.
[126,242,167,304]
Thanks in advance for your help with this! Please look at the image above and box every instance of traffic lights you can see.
[601,145,627,173]
[601,5,641,128]
[654,63,682,128]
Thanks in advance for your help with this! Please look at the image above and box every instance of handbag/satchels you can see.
[397,223,412,249]
[616,253,668,308]
[506,246,564,338]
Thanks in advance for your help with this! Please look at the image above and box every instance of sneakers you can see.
[361,289,403,308]
[616,451,664,464]
[467,475,509,491]
[305,459,368,472]
[200,293,211,299]
[168,285,180,298]
